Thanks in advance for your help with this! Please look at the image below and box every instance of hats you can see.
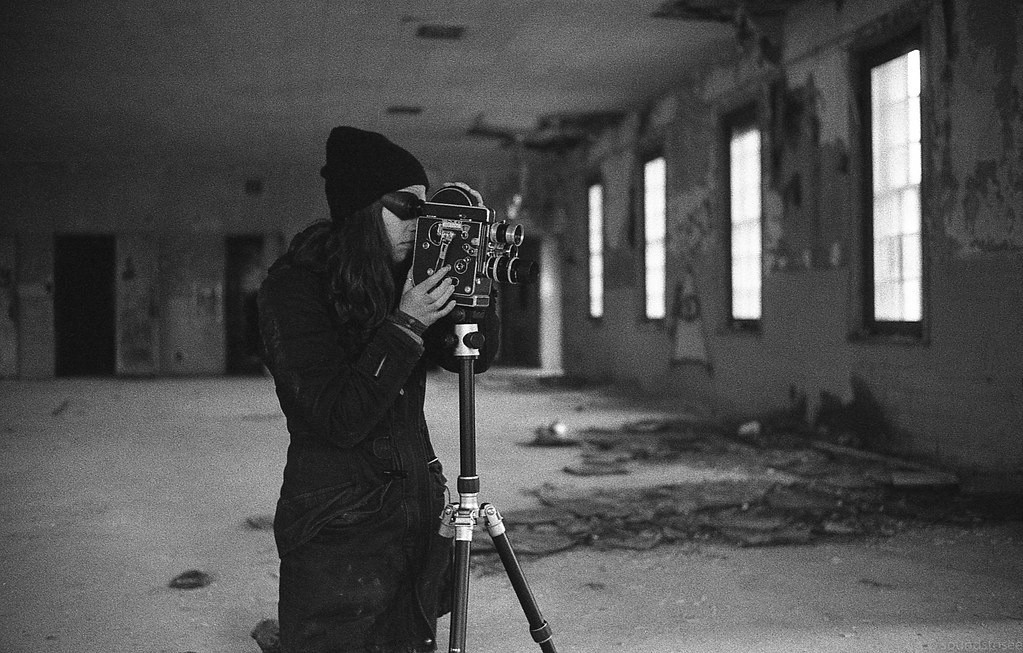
[319,124,431,223]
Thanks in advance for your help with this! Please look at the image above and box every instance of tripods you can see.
[364,322,557,653]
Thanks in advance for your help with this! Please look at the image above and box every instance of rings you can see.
[406,277,413,281]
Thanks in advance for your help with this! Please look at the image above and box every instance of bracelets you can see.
[387,308,428,336]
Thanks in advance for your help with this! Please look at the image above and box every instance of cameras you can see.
[409,182,539,312]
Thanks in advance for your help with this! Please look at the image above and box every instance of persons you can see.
[258,126,502,653]
